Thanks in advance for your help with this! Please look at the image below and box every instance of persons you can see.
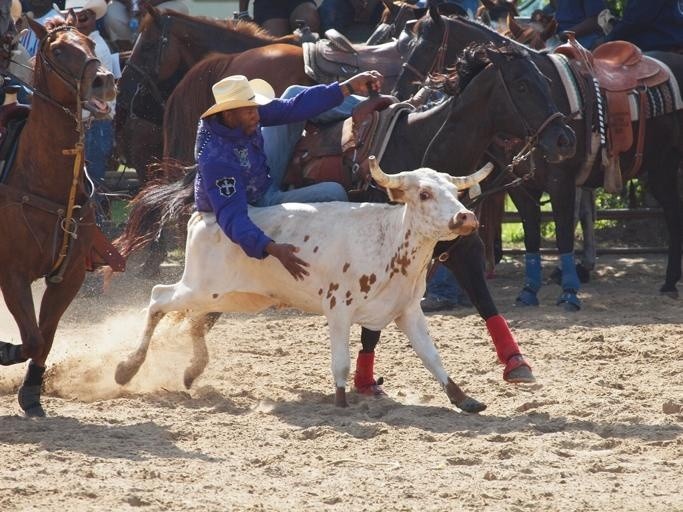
[53,0,118,236]
[419,262,473,312]
[192,69,383,280]
[2,0,680,85]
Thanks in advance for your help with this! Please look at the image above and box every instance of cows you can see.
[115,156,494,413]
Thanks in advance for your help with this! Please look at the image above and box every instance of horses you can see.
[0,9,118,418]
[115,0,683,398]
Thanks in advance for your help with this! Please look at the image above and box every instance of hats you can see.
[53,0,108,20]
[200,75,276,120]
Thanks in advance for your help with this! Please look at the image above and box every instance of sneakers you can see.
[420,298,452,312]
